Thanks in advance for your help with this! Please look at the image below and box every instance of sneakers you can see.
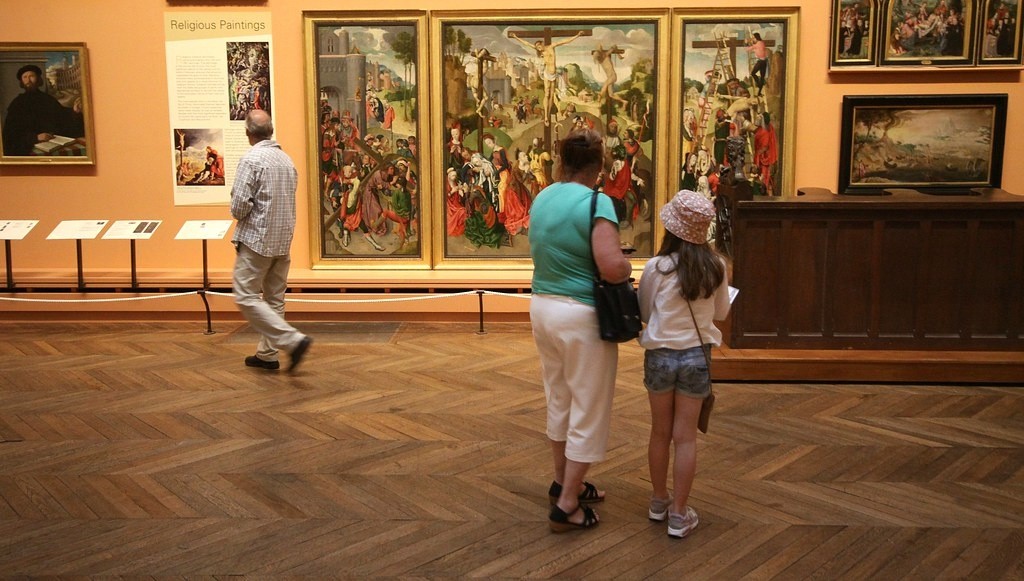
[666,504,699,538]
[648,488,673,520]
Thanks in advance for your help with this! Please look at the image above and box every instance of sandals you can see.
[549,479,606,505]
[548,503,601,532]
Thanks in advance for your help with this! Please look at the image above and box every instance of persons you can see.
[527,127,631,533]
[636,190,732,539]
[230,109,314,372]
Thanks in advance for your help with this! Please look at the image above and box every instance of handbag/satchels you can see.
[698,394,715,435]
[592,277,644,344]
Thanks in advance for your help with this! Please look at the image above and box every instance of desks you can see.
[715,178,1024,354]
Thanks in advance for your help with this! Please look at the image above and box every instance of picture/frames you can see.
[300,8,433,272]
[0,41,96,167]
[667,5,803,253]
[429,5,672,273]
[825,0,1024,75]
[837,92,1010,197]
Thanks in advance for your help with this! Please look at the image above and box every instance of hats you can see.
[658,190,714,244]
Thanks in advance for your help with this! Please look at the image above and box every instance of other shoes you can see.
[244,353,280,371]
[284,335,312,371]
